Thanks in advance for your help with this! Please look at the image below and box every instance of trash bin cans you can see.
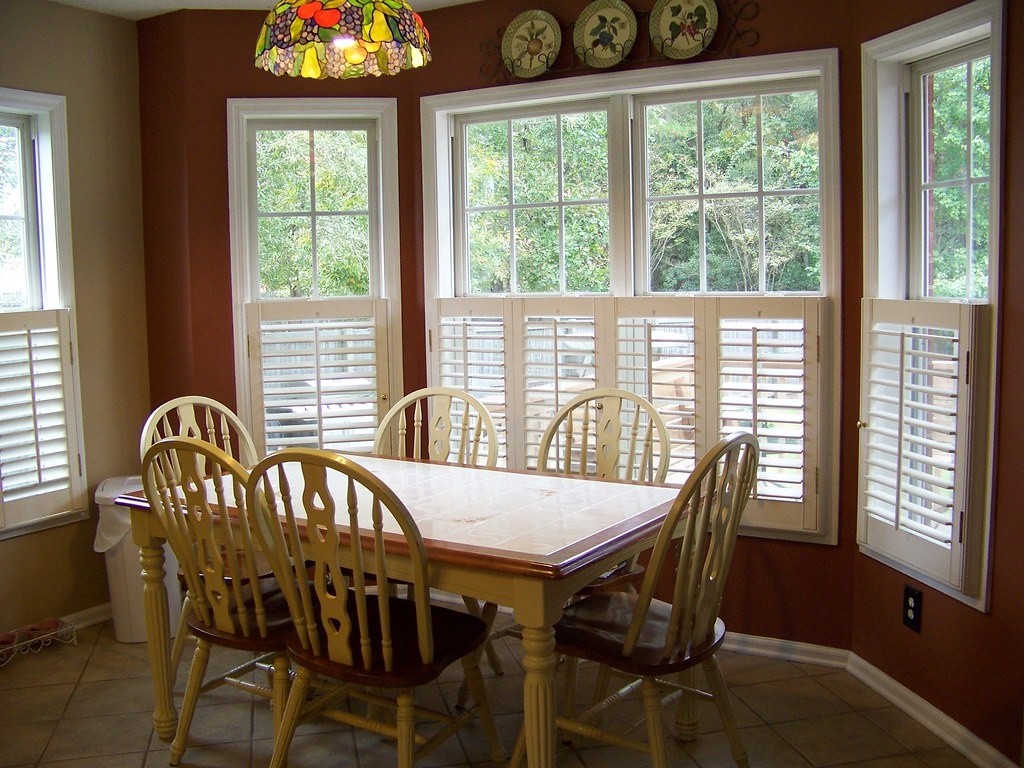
[95,475,184,643]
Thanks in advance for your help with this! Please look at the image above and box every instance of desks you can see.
[114,448,718,768]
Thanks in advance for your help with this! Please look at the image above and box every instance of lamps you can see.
[254,0,433,81]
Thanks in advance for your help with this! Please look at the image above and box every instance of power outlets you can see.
[902,583,923,634]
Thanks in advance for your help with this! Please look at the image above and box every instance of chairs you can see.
[507,430,760,768]
[245,447,505,768]
[138,395,315,685]
[325,387,504,675]
[142,436,352,768]
[453,387,671,725]
